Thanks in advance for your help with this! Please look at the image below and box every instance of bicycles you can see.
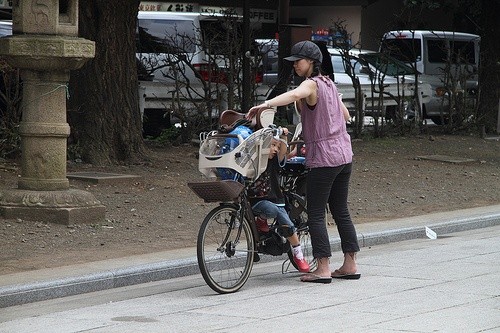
[188,110,326,294]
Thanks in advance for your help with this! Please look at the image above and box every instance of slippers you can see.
[301,273,331,283]
[331,270,361,279]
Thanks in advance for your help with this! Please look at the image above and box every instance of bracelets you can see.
[264,100,272,108]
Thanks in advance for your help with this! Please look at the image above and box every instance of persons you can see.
[246,40,361,283]
[247,127,310,273]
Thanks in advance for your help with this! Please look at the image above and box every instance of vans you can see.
[378,30,482,126]
[135,11,244,130]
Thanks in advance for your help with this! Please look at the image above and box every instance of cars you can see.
[253,46,432,130]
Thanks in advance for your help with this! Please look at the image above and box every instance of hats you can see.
[283,40,322,65]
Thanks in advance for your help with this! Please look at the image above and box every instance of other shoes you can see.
[256,217,269,232]
[293,253,310,272]
